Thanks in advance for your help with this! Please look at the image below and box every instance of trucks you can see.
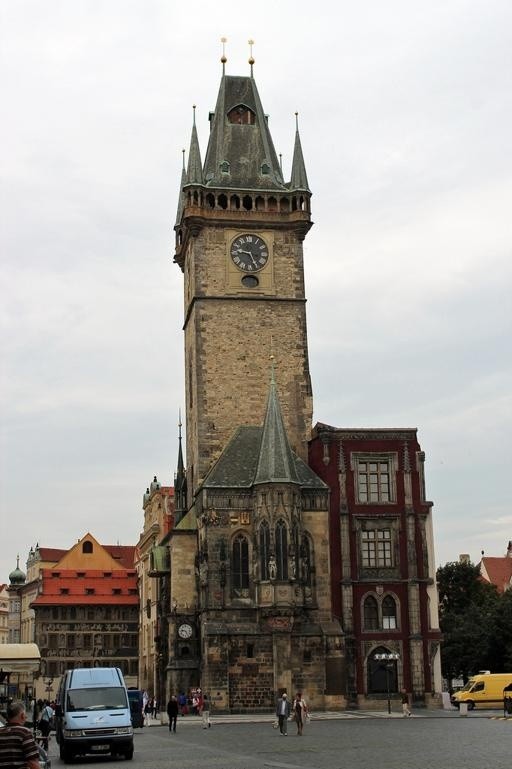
[56,666,144,764]
[450,669,512,711]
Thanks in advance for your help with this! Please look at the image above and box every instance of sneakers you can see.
[280,732,287,736]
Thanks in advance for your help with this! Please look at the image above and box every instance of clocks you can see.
[177,623,193,639]
[230,233,270,273]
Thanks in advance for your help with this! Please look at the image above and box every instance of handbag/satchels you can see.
[304,712,310,725]
[272,721,279,729]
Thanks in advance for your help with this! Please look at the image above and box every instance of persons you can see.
[276,692,308,737]
[401,687,412,718]
[0,698,55,769]
[144,692,212,733]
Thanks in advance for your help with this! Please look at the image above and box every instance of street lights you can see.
[374,650,399,713]
